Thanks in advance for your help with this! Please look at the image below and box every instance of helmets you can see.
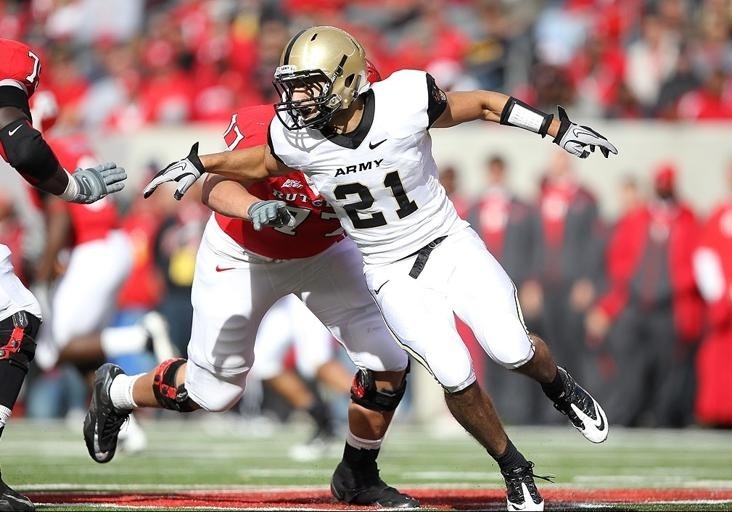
[273,26,370,130]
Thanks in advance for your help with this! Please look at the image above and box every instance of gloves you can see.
[248,200,297,232]
[143,142,206,200]
[58,163,127,204]
[553,105,618,158]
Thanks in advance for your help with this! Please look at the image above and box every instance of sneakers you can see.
[84,362,134,462]
[331,463,421,508]
[0,480,35,512]
[553,366,608,443]
[501,461,555,512]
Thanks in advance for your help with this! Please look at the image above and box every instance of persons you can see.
[143,25,617,511]
[84,60,422,508]
[1,39,126,511]
[1,0,732,463]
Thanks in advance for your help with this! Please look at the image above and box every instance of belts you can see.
[391,236,447,279]
